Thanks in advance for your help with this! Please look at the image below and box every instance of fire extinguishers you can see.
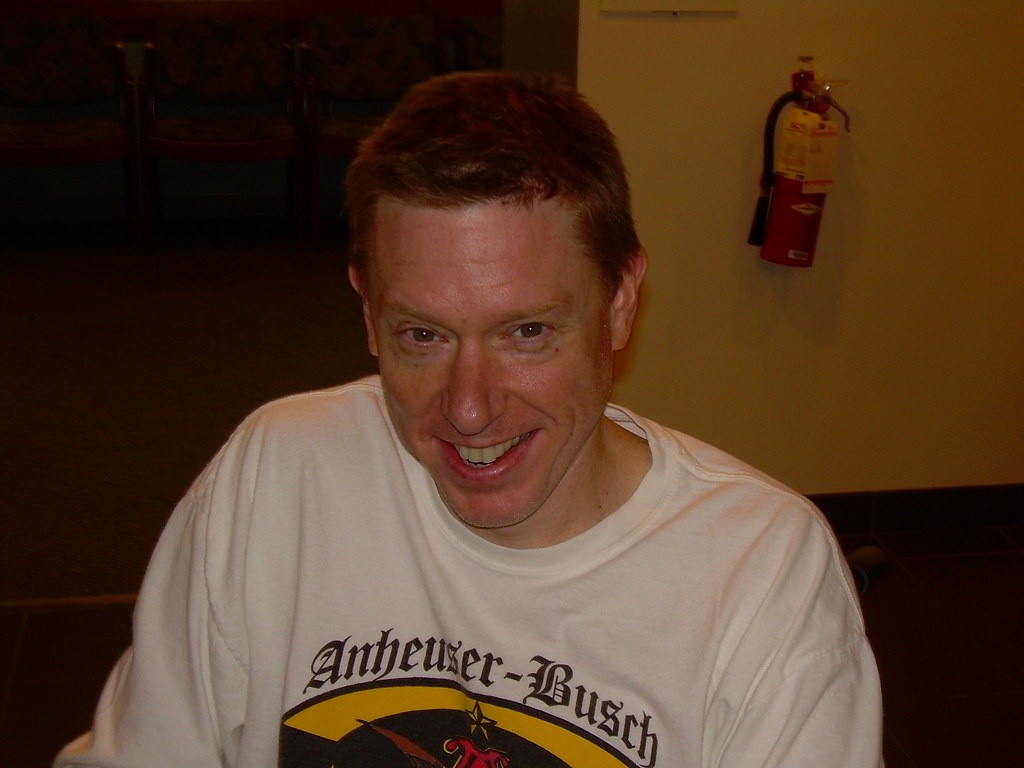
[747,73,852,270]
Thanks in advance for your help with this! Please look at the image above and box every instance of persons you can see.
[52,72,885,768]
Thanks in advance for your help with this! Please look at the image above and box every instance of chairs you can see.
[0,14,503,257]
